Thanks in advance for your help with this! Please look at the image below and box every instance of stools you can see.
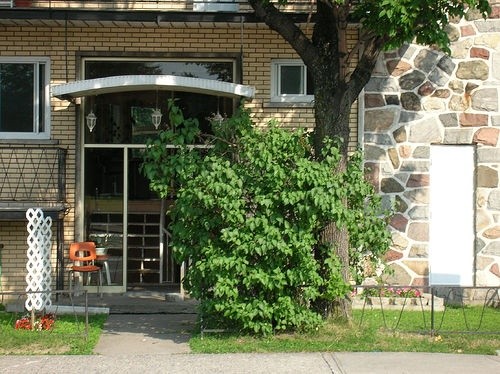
[85,254,113,286]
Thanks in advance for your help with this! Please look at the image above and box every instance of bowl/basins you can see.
[96,248,108,255]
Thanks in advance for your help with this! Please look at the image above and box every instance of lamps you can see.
[86,95,98,132]
[152,87,163,131]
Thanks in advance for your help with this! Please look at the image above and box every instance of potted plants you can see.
[94,243,104,254]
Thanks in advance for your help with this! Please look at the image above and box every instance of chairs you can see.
[66,241,103,302]
